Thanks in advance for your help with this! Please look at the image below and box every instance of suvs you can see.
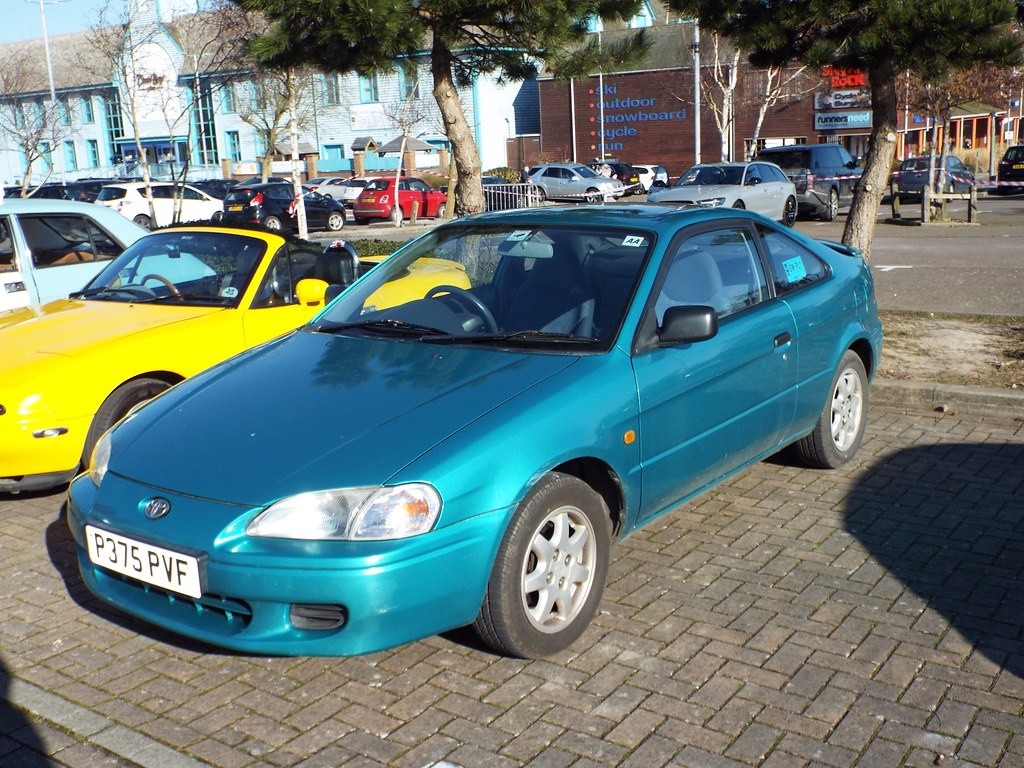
[752,143,866,221]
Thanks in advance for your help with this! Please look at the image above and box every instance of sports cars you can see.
[0,227,473,500]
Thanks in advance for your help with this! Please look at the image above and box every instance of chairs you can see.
[503,265,586,333]
[225,249,274,305]
[616,248,731,327]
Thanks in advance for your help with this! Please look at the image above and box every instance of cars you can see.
[305,176,350,206]
[586,161,641,198]
[90,182,226,226]
[438,175,528,210]
[0,197,218,309]
[888,155,976,204]
[996,145,1024,193]
[647,161,800,227]
[631,165,668,193]
[223,181,347,232]
[62,202,883,659]
[350,176,450,225]
[6,176,292,203]
[525,162,624,204]
[344,175,382,208]
